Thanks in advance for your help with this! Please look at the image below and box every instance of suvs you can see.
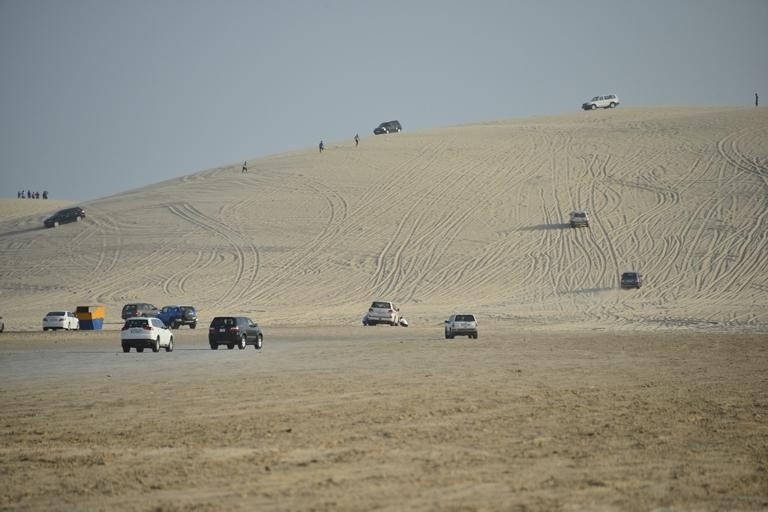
[373,120,402,134]
[121,317,173,352]
[570,211,588,228]
[368,301,399,326]
[155,305,198,329]
[209,317,263,349]
[583,94,620,110]
[621,273,642,288]
[445,314,478,339]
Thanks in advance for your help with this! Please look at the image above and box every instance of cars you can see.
[122,303,158,319]
[43,312,80,330]
[44,208,86,227]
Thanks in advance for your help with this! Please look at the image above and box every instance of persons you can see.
[17,190,49,199]
[353,133,361,147]
[753,93,759,106]
[318,140,325,152]
[241,160,248,174]
